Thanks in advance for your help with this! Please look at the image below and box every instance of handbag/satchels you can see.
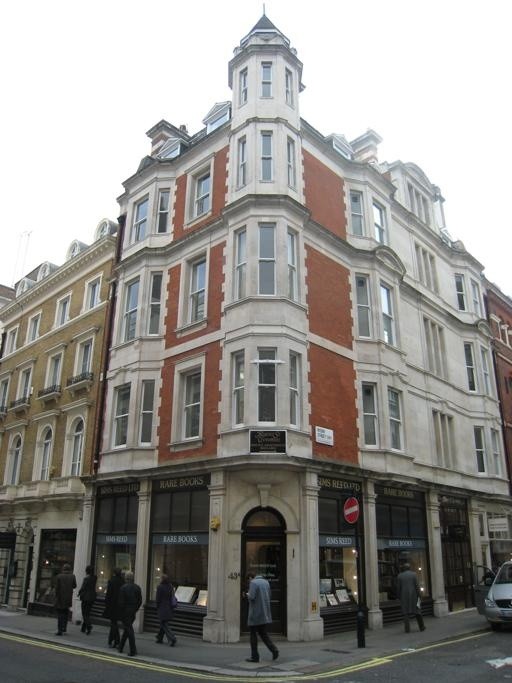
[170,588,177,608]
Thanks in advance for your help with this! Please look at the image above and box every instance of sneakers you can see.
[245,657,259,662]
[273,649,278,660]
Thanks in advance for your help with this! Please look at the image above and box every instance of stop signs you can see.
[343,496,361,525]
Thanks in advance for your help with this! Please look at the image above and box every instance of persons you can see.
[244,571,279,663]
[53,563,77,635]
[396,563,426,632]
[116,570,142,656]
[103,568,125,648]
[77,566,97,635]
[155,574,177,646]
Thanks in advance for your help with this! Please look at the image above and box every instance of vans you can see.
[320,559,396,596]
[473,559,512,629]
